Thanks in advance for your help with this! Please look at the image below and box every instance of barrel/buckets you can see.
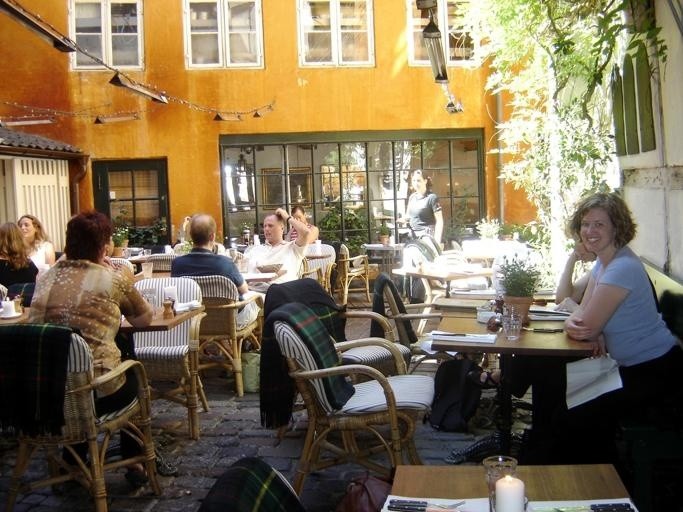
[124,463,149,484]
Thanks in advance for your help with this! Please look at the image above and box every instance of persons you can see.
[19,216,56,279]
[565,192,681,461]
[479,240,598,464]
[285,206,319,246]
[171,215,266,378]
[0,223,38,286]
[243,207,310,286]
[28,210,160,494]
[396,170,444,304]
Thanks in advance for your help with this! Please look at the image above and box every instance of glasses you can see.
[109,72,169,105]
[253,105,274,118]
[213,113,244,121]
[0,0,76,54]
[237,146,247,172]
[416,0,464,114]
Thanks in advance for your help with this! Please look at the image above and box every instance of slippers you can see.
[427,351,483,434]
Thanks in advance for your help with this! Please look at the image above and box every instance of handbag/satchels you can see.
[286,216,292,223]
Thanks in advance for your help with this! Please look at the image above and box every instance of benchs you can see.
[604,255,683,512]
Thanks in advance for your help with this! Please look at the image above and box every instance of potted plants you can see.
[500,223,514,240]
[379,223,392,245]
[495,251,542,324]
[117,226,129,247]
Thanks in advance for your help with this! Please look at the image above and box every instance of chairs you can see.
[0,232,442,511]
[451,240,489,268]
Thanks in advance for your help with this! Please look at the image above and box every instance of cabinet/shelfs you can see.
[18,297,25,313]
[163,300,174,319]
[13,298,22,314]
[164,296,177,316]
[242,226,250,245]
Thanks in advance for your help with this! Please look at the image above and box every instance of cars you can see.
[164,245,171,255]
[144,249,152,256]
[145,289,156,320]
[2,300,14,316]
[141,262,153,279]
[122,249,132,259]
[501,314,522,340]
[502,302,520,319]
[483,456,518,512]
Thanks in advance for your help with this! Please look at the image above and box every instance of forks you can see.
[522,326,564,333]
[388,497,466,512]
[553,501,634,512]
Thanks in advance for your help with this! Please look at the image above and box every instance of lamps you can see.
[451,240,489,268]
[253,105,274,118]
[0,0,76,54]
[213,113,244,121]
[109,72,169,105]
[237,146,247,172]
[416,0,464,114]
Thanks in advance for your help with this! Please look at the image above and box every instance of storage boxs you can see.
[604,255,683,512]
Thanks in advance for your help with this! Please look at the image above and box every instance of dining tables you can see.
[117,226,129,247]
[500,223,514,240]
[495,251,542,324]
[379,223,392,245]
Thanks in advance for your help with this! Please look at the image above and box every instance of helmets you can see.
[256,264,284,273]
[131,248,143,256]
[0,309,23,319]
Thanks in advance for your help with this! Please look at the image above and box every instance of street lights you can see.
[242,348,261,393]
[335,469,392,512]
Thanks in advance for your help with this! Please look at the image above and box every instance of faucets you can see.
[468,369,500,389]
